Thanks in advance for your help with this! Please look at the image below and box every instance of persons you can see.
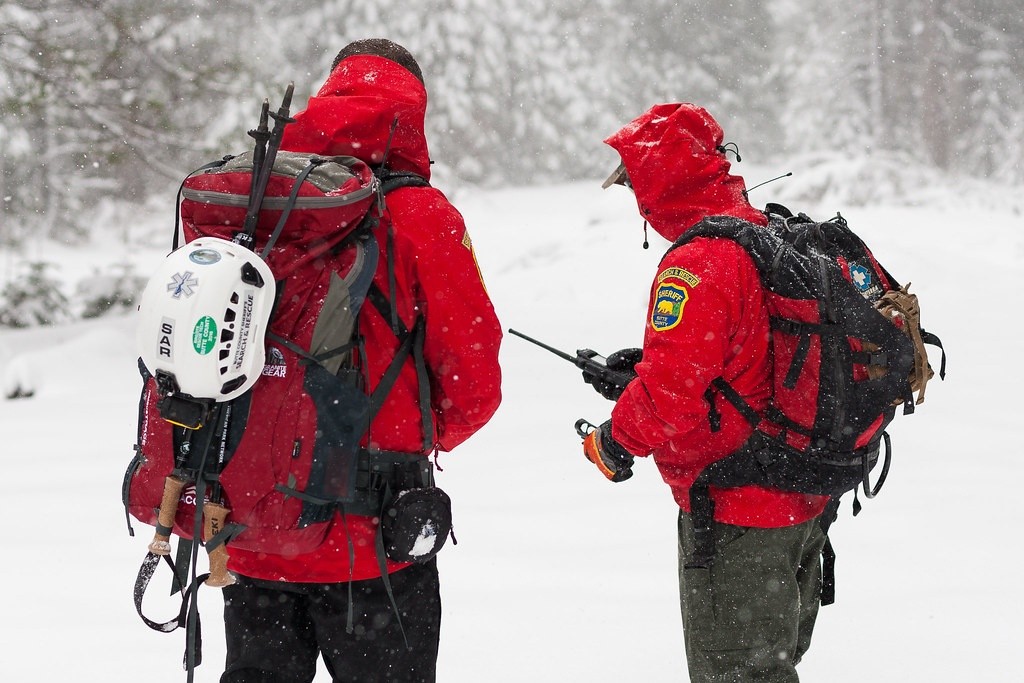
[218,39,506,683]
[581,103,844,683]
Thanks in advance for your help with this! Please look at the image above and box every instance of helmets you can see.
[136,235,276,405]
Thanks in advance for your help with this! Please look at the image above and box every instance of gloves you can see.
[577,346,644,403]
[579,417,637,487]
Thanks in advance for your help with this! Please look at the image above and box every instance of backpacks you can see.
[654,204,949,610]
[118,147,448,679]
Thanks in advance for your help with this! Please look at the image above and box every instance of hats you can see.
[600,157,631,196]
